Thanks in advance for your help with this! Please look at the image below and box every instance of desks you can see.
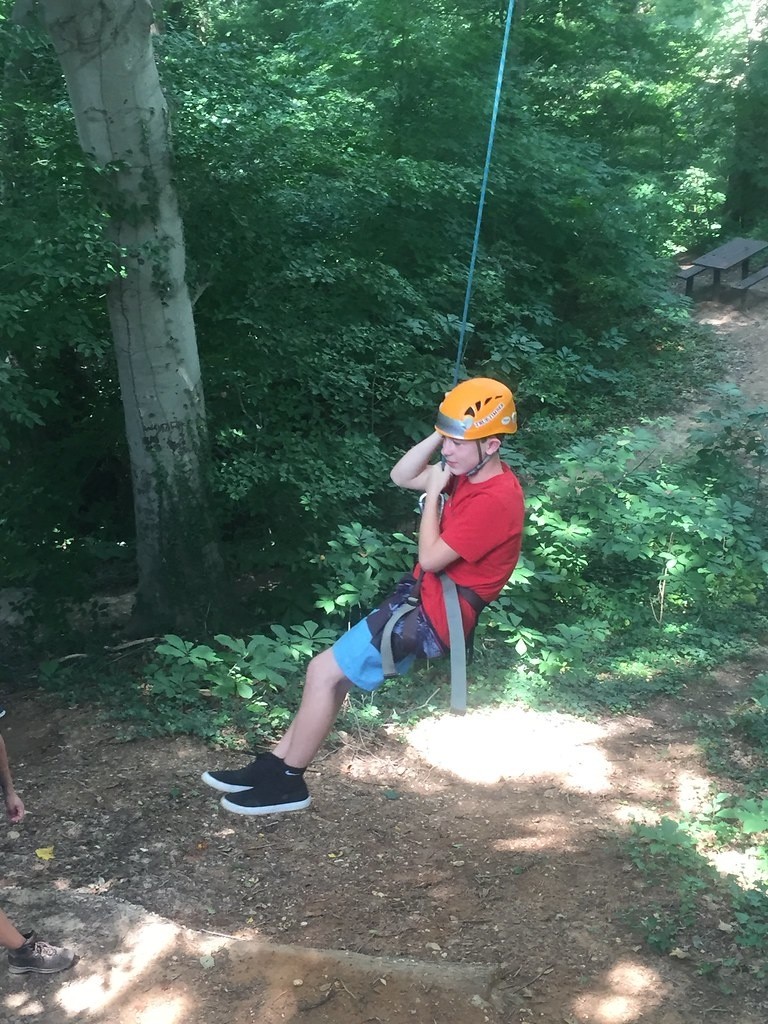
[692,237,768,302]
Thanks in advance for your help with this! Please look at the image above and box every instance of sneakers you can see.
[7,930,75,975]
[200,751,311,815]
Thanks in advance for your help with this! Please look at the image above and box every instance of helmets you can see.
[434,377,518,440]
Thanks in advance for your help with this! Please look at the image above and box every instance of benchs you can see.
[676,264,709,297]
[731,267,768,302]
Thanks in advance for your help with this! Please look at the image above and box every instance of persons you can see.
[201,378,524,816]
[0,735,75,973]
